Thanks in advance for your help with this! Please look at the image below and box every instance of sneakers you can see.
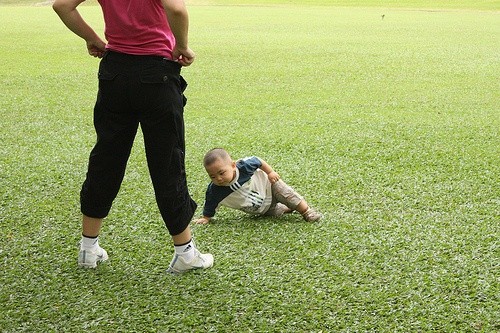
[78,245,109,268]
[167,248,214,275]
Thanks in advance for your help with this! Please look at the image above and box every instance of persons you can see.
[192,148,322,224]
[52,0,214,273]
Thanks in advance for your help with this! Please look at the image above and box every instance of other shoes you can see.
[301,206,321,223]
